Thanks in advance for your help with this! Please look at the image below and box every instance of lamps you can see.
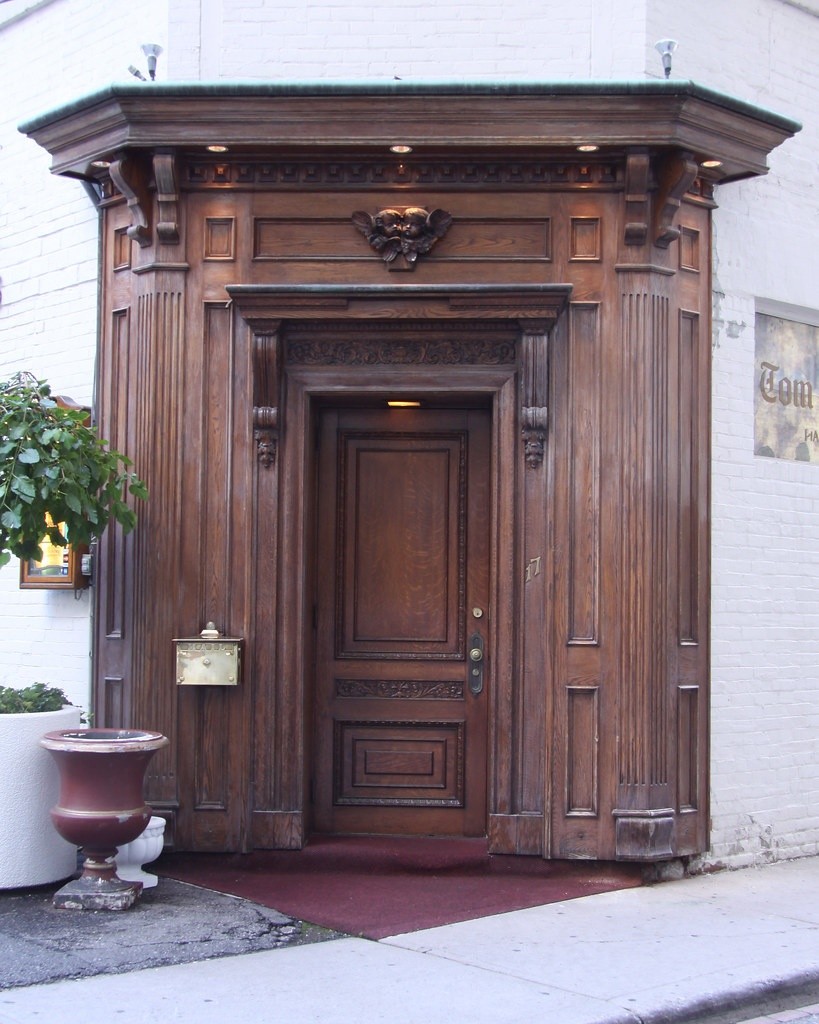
[127,42,164,82]
[653,39,679,80]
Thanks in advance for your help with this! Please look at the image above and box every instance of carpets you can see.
[145,836,725,941]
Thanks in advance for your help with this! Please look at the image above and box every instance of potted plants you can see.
[0,681,94,889]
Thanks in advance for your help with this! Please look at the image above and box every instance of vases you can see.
[116,816,166,889]
[39,727,171,911]
